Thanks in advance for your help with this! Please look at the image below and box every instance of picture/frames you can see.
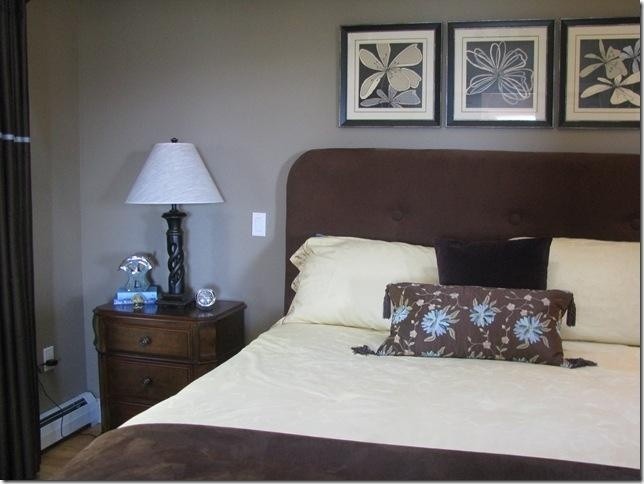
[447,19,554,127]
[336,21,442,125]
[559,17,642,126]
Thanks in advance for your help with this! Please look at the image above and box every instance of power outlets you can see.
[42,344,55,371]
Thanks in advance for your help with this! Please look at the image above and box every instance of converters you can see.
[46,359,58,366]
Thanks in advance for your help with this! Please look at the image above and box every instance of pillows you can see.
[281,232,640,369]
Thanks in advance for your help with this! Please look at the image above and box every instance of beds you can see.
[46,147,640,481]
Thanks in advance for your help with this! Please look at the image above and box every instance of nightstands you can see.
[91,300,248,433]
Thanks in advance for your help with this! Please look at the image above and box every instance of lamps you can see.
[124,138,225,311]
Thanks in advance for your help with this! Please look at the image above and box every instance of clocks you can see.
[192,286,216,311]
